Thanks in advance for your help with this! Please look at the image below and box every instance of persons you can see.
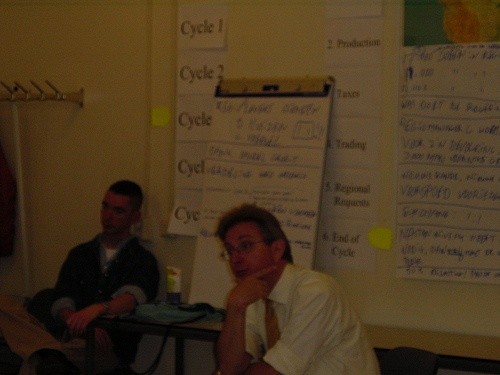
[213,203,382,375]
[0,179,161,375]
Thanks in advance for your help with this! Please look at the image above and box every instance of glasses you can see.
[219,240,263,261]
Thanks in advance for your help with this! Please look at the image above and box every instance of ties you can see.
[264,300,280,349]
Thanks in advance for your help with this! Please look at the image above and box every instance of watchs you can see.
[102,301,112,314]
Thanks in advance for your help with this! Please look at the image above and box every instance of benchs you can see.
[372,347,500,375]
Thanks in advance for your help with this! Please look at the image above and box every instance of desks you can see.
[86,315,224,375]
